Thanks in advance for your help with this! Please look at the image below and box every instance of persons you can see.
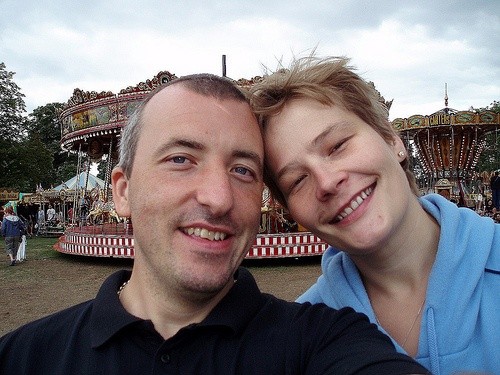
[474,177,485,214]
[46,205,55,221]
[67,206,75,224]
[0,206,27,266]
[36,207,45,237]
[0,73,434,375]
[489,171,500,212]
[248,44,500,375]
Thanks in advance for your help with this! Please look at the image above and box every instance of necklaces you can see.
[375,298,426,348]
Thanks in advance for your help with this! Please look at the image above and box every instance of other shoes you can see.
[10,259,15,266]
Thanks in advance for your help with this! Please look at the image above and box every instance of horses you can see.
[85,182,124,223]
